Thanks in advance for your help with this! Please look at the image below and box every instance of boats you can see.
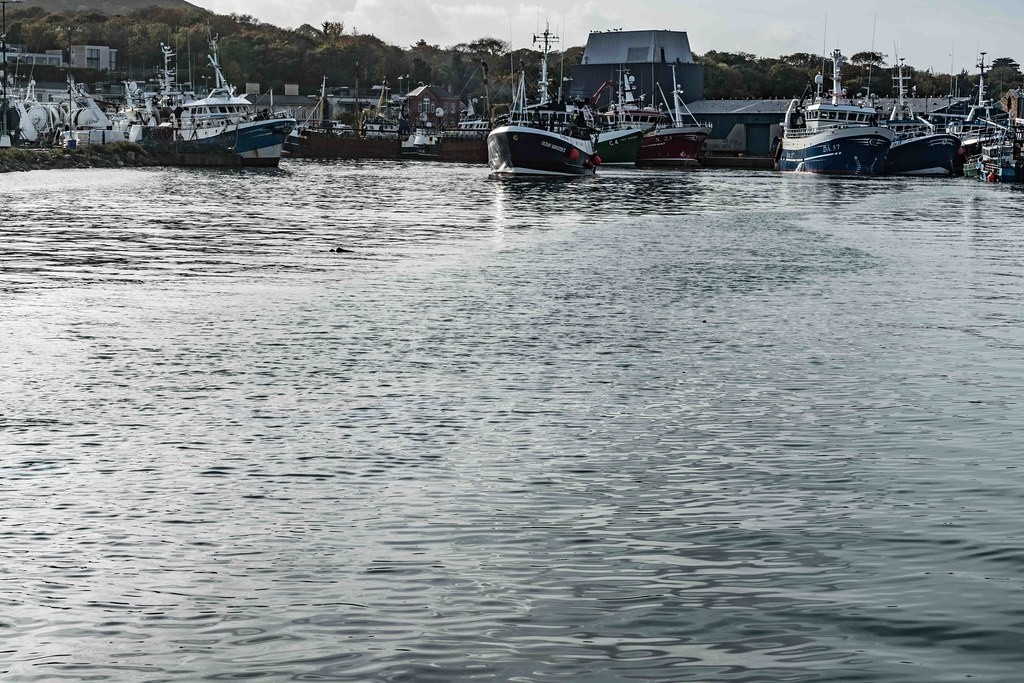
[487,102,601,178]
[588,96,645,167]
[946,45,1024,182]
[780,10,897,178]
[634,64,714,167]
[0,23,298,168]
[881,41,964,175]
[276,58,507,163]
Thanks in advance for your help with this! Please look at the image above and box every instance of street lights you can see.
[2,0,24,137]
[397,75,404,93]
[406,73,410,120]
